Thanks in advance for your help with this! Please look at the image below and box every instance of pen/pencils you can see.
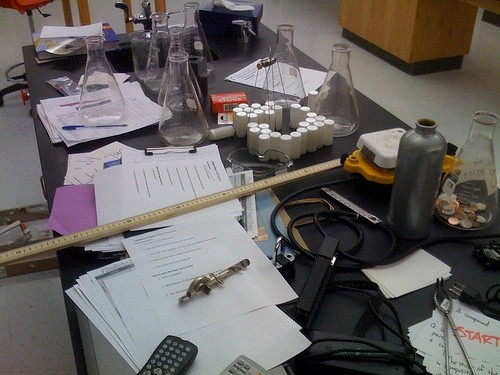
[61,123,127,130]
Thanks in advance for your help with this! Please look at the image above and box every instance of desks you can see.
[21,21,500,375]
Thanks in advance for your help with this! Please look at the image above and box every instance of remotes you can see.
[136,334,198,375]
[217,354,267,375]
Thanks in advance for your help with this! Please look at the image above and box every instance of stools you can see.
[0,0,54,117]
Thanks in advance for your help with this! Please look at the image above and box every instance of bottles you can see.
[261,24,306,110]
[388,118,447,237]
[439,111,499,230]
[145,14,168,92]
[316,43,359,137]
[80,36,124,124]
[159,52,208,146]
[158,26,203,112]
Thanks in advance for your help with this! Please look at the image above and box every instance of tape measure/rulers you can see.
[1,151,343,264]
[320,184,386,232]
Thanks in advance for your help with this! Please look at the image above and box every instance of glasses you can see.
[226,148,294,178]
